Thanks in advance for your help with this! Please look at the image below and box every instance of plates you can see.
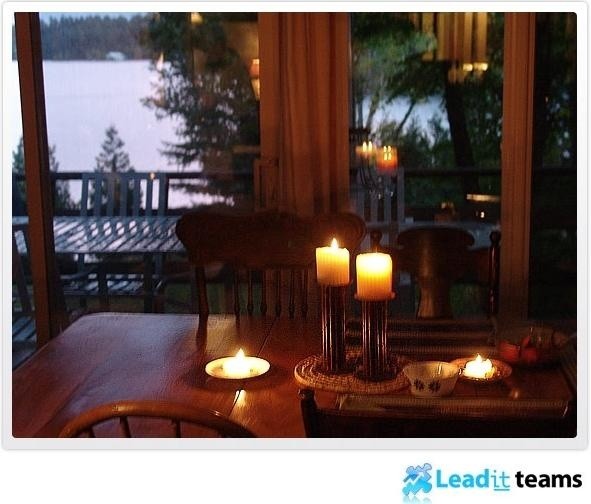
[204,356,271,381]
[450,357,513,386]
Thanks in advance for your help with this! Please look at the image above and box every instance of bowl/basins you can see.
[403,361,461,399]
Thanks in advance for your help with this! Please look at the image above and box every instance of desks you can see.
[14,313,577,436]
[12,215,188,310]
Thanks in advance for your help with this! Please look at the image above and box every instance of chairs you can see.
[11,225,82,346]
[369,225,501,321]
[174,206,366,316]
[77,172,168,312]
[60,402,254,438]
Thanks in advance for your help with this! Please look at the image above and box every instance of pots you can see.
[497,325,576,369]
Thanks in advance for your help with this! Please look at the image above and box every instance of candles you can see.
[222,350,251,378]
[356,252,393,300]
[314,238,349,286]
[376,146,397,170]
[463,354,493,378]
